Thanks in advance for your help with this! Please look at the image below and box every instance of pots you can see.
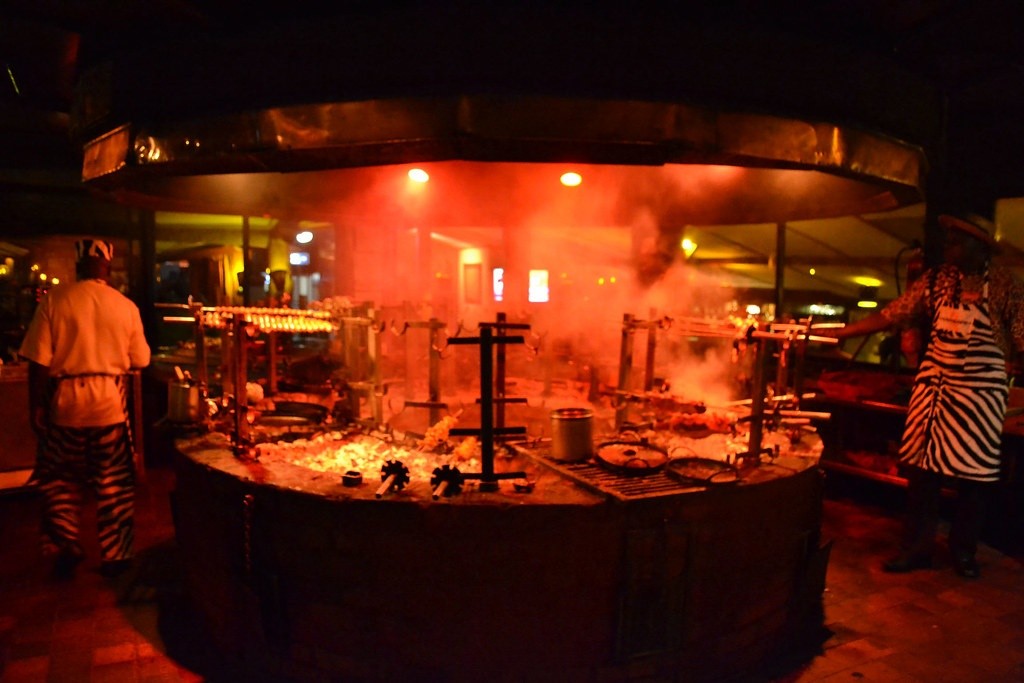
[596,428,669,473]
[665,446,740,486]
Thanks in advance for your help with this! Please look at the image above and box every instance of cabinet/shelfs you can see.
[807,361,915,488]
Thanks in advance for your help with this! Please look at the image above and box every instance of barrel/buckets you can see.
[550,406,595,462]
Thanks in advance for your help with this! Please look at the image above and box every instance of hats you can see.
[76,237,113,261]
[939,209,1012,252]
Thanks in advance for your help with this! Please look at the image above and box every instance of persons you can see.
[816,208,1024,579]
[17,239,151,579]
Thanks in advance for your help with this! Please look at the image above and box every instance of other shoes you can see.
[55,544,86,571]
[955,555,979,579]
[103,558,138,577]
[885,551,934,575]
